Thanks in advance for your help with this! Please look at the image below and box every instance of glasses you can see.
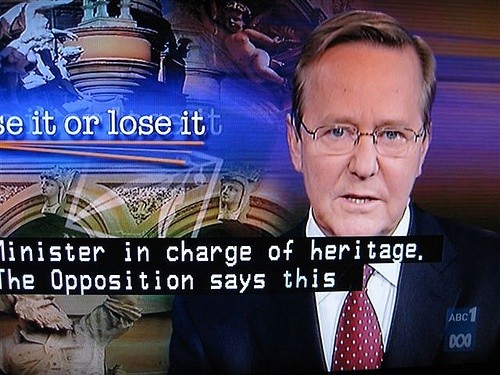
[297,121,427,159]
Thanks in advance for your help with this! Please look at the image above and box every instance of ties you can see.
[331,265,386,373]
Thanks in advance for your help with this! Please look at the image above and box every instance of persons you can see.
[217,166,262,223]
[40,163,77,214]
[1,293,142,374]
[202,0,285,90]
[169,9,499,374]
